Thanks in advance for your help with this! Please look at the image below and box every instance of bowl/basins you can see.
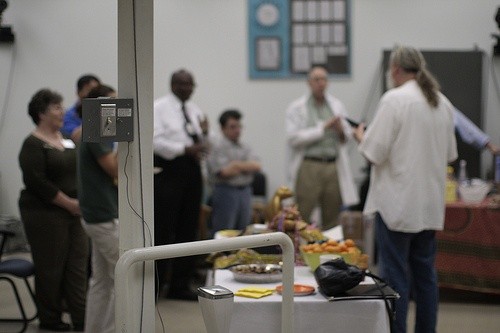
[300,244,361,272]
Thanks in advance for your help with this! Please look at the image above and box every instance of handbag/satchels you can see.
[313,257,397,321]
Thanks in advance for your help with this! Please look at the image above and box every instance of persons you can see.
[351,45,459,333]
[153,69,212,301]
[74,85,119,333]
[206,110,262,235]
[285,67,355,234]
[60,74,101,291]
[18,88,90,333]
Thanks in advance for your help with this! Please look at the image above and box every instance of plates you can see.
[275,284,315,296]
[229,263,284,283]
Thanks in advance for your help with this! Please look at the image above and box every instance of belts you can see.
[221,186,252,191]
[304,157,336,162]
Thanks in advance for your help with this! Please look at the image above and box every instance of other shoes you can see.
[167,284,198,299]
[40,321,70,330]
[73,323,84,333]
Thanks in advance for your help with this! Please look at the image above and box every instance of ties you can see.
[182,105,199,144]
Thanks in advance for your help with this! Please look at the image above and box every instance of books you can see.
[318,283,400,301]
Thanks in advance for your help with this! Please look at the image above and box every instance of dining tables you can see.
[207,230,390,333]
[436,195,500,294]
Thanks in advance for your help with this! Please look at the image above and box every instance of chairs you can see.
[0,230,39,333]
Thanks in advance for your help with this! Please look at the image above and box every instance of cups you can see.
[459,180,490,203]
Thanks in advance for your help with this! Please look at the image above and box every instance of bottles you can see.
[456,159,469,189]
[445,166,456,203]
